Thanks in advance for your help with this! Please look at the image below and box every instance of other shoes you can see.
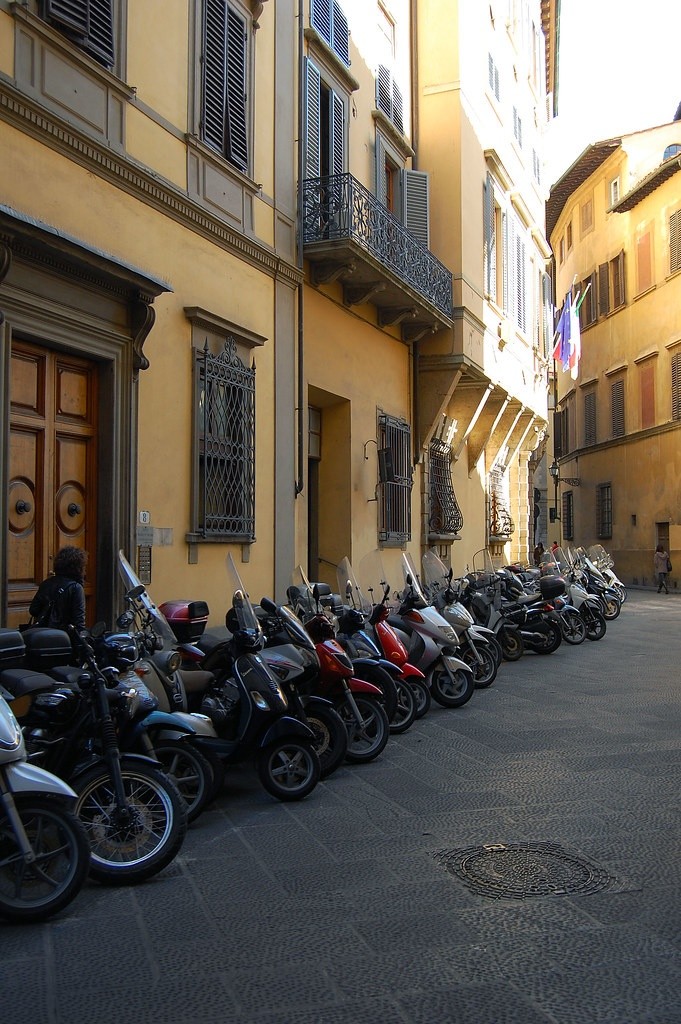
[665,590,669,594]
[657,589,661,593]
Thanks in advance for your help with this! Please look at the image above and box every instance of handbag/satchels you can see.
[667,557,673,572]
[19,617,42,633]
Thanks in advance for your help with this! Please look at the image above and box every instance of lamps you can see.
[549,461,581,486]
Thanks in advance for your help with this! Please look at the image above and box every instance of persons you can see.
[29,546,87,639]
[551,541,560,557]
[654,543,669,594]
[534,542,544,563]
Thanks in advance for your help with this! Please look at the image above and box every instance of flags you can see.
[554,302,577,369]
[570,309,581,381]
[556,293,571,372]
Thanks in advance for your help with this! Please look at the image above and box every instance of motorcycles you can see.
[0,543,627,926]
